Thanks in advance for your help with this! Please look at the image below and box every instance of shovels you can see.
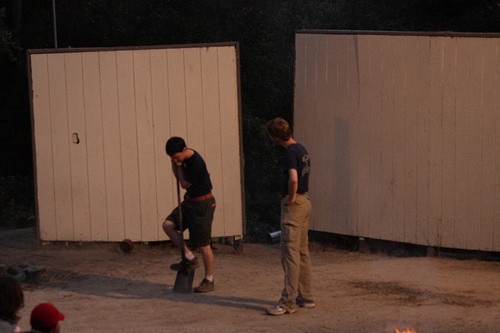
[171,172,196,295]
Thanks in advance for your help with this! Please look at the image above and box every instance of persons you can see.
[162,136,216,294]
[18,303,65,333]
[0,275,27,333]
[267,116,317,317]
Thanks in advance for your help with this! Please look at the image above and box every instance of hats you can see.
[30,302,64,327]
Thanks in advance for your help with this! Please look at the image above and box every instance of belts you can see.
[191,192,214,201]
[284,192,305,195]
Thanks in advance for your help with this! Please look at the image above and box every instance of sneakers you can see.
[296,296,316,307]
[194,278,215,292]
[170,256,200,270]
[266,299,298,315]
